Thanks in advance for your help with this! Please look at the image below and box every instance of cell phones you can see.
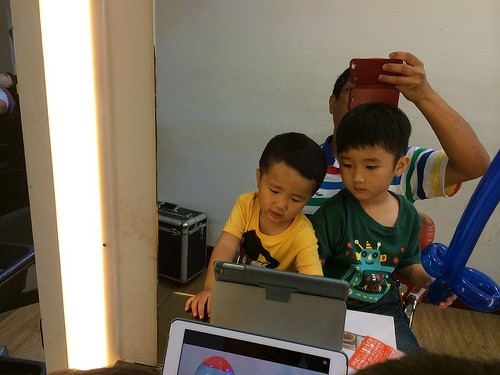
[350,58,405,84]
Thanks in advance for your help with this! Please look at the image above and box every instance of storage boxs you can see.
[158,202,209,286]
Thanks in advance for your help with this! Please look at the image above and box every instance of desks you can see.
[1,263,44,366]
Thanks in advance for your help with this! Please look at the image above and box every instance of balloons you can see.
[421,146,500,313]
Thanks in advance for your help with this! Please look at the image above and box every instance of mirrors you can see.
[156,0,500,375]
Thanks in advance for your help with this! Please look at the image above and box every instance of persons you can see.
[310,102,453,354]
[0,72,15,114]
[184,132,323,319]
[300,51,492,225]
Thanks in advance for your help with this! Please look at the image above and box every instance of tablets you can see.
[160,318,349,375]
[208,260,349,352]
